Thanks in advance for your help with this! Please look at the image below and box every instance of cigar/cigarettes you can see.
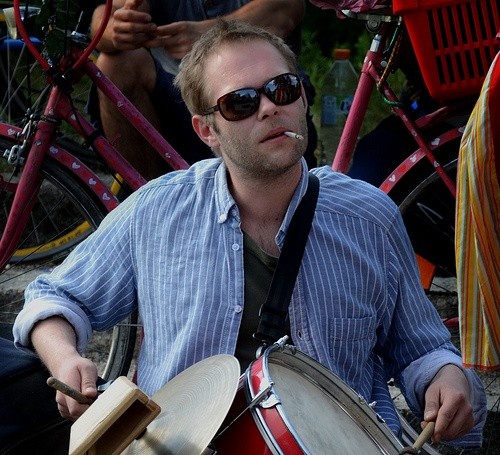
[284,131,304,140]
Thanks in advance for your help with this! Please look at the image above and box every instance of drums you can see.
[205,344,406,455]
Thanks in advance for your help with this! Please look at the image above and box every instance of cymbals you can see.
[120,354,241,455]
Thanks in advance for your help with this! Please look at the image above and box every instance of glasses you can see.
[198,73,302,121]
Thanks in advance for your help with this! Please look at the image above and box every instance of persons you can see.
[90,0,303,190]
[13,16,476,446]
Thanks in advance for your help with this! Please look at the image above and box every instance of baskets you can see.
[393,0,500,101]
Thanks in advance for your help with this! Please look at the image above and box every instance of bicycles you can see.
[0,0,500,455]
